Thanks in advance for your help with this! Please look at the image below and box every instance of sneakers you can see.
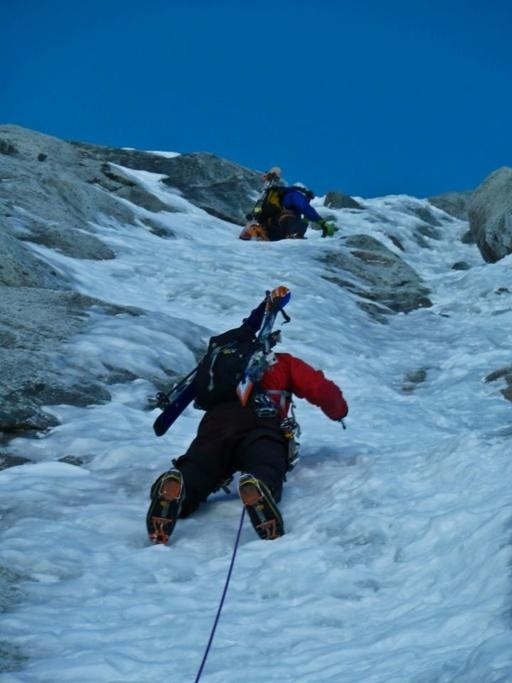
[146,468,188,544]
[237,473,284,540]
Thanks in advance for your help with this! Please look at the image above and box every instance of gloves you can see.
[319,219,340,237]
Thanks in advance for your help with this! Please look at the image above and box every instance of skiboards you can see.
[239,167,280,240]
[154,286,290,436]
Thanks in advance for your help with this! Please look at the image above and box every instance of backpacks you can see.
[252,185,303,218]
[194,329,262,408]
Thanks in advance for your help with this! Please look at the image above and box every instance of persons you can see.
[238,180,341,240]
[143,351,348,540]
